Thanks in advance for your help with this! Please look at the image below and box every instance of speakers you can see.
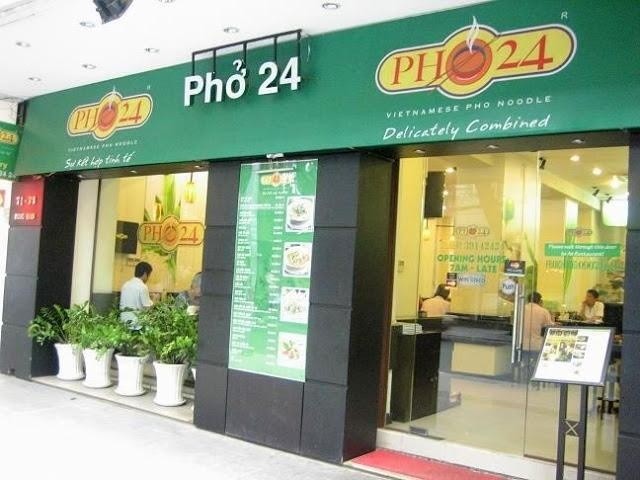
[116,220,139,254]
[425,171,444,217]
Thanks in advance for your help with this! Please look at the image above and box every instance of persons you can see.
[521,291,553,362]
[420,284,450,317]
[175,272,202,310]
[119,262,153,335]
[577,289,604,322]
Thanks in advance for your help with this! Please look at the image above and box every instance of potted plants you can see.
[26,296,199,408]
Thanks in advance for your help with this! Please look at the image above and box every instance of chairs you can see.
[601,302,623,335]
